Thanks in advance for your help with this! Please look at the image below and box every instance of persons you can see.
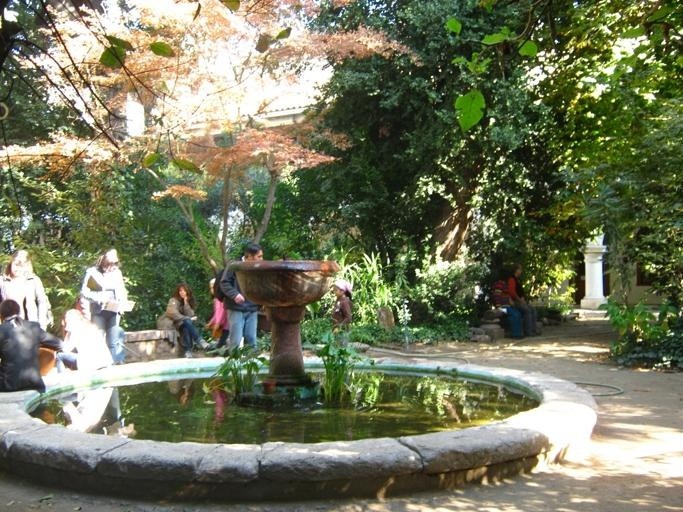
[492,265,520,340]
[506,264,543,337]
[157,285,209,357]
[331,280,352,346]
[218,243,264,353]
[0,246,132,395]
[203,277,229,352]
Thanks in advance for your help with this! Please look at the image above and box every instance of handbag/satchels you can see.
[213,268,226,302]
[157,314,175,329]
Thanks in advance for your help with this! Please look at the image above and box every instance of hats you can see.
[331,279,348,292]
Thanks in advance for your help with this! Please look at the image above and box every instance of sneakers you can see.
[201,340,212,350]
[185,351,193,358]
